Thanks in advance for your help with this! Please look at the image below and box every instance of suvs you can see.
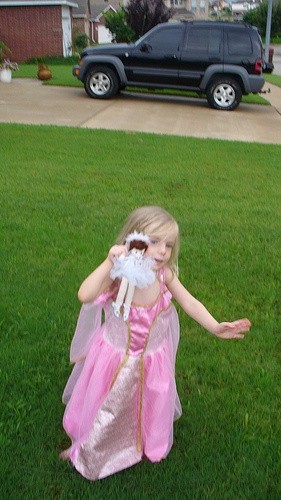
[71,19,266,110]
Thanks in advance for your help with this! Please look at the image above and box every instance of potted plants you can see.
[0,59,18,83]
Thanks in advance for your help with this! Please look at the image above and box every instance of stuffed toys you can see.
[110,230,155,321]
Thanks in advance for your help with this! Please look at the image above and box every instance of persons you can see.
[59,205,251,481]
